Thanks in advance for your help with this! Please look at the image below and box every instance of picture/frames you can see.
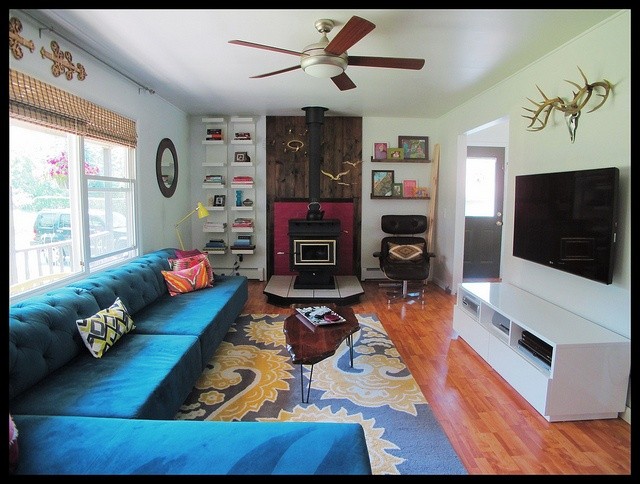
[372,169,394,197]
[213,195,225,206]
[394,182,403,198]
[398,136,429,160]
[373,143,389,160]
[402,179,418,197]
[235,152,247,162]
[387,148,404,160]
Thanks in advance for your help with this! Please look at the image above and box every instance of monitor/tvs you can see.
[514,167,618,285]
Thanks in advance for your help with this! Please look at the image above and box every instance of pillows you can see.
[76,297,136,358]
[168,252,214,284]
[174,248,202,260]
[161,261,213,297]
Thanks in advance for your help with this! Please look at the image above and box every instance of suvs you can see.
[29,206,127,266]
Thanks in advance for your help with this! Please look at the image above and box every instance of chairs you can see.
[379,215,435,303]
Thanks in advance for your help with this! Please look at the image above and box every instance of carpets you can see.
[169,313,470,477]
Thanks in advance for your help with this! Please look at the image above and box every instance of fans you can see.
[228,15,425,91]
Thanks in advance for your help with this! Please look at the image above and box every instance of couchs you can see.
[11,412,371,475]
[9,286,202,420]
[70,246,248,370]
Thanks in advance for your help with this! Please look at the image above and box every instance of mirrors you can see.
[156,137,178,198]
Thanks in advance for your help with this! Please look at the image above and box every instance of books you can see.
[203,222,227,228]
[232,176,253,183]
[230,235,256,249]
[232,217,253,226]
[203,174,222,184]
[203,242,227,250]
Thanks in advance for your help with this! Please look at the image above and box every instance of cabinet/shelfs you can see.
[453,282,631,422]
